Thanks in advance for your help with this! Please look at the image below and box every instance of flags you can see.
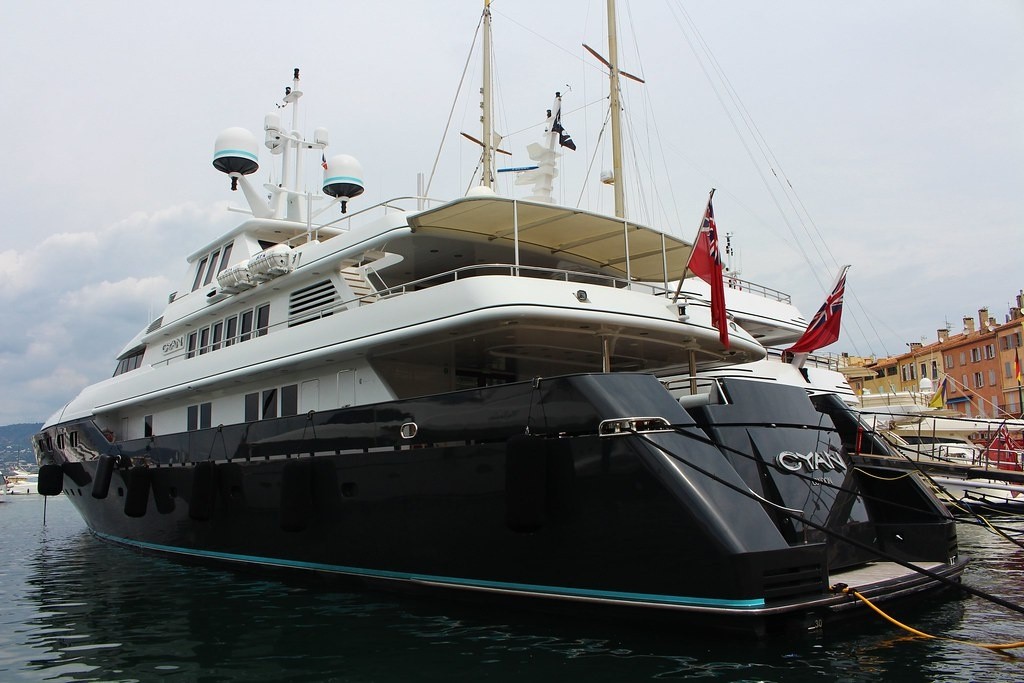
[782,265,850,364]
[321,149,328,172]
[982,423,1024,498]
[928,375,946,408]
[1016,352,1023,387]
[551,108,577,151]
[684,189,729,352]
[937,382,941,388]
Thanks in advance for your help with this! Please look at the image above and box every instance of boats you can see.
[5,473,39,494]
[29,0,963,633]
[855,386,1015,513]
